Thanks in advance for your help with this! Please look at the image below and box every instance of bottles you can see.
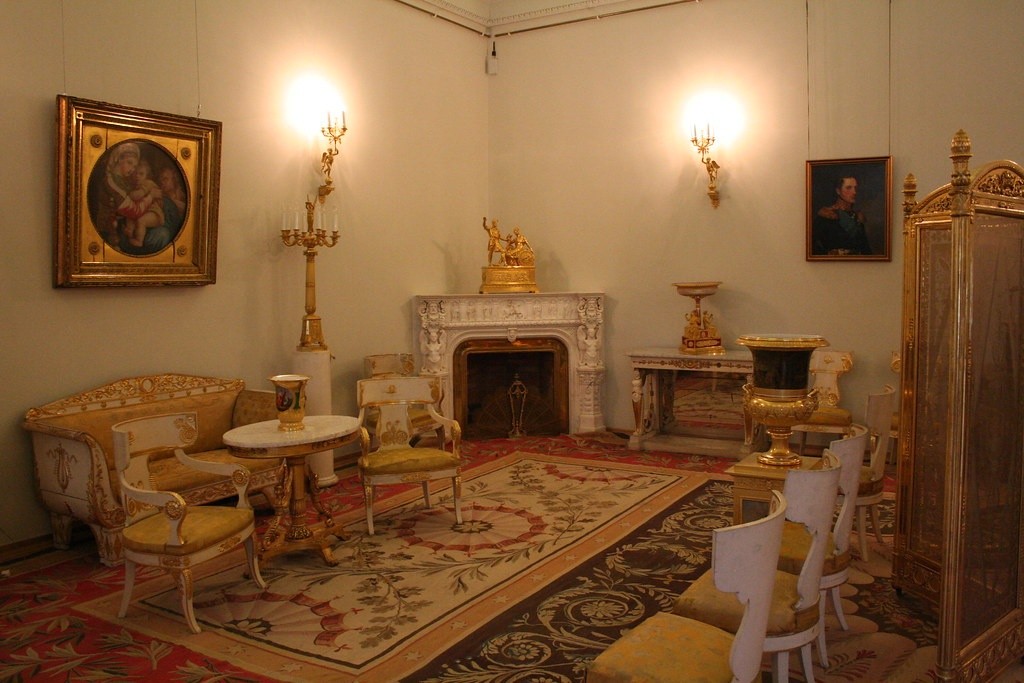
[268,373,311,431]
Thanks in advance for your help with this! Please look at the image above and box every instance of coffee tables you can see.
[223,415,363,579]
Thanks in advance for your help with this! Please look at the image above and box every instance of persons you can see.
[814,174,871,256]
[90,141,187,255]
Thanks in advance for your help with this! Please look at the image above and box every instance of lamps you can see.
[691,123,719,209]
[281,195,340,355]
[317,109,350,204]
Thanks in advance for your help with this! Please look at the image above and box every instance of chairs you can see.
[672,449,842,683]
[779,422,868,631]
[586,490,788,683]
[356,374,463,535]
[363,353,446,451]
[110,410,267,633]
[834,384,898,559]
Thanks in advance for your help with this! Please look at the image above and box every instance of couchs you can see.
[24,373,280,569]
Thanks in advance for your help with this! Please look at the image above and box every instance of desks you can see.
[726,452,822,526]
[792,422,852,455]
[626,346,856,459]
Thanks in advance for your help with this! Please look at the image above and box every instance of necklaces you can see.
[832,205,859,231]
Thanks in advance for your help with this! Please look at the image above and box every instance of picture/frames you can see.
[54,94,222,287]
[805,155,892,261]
